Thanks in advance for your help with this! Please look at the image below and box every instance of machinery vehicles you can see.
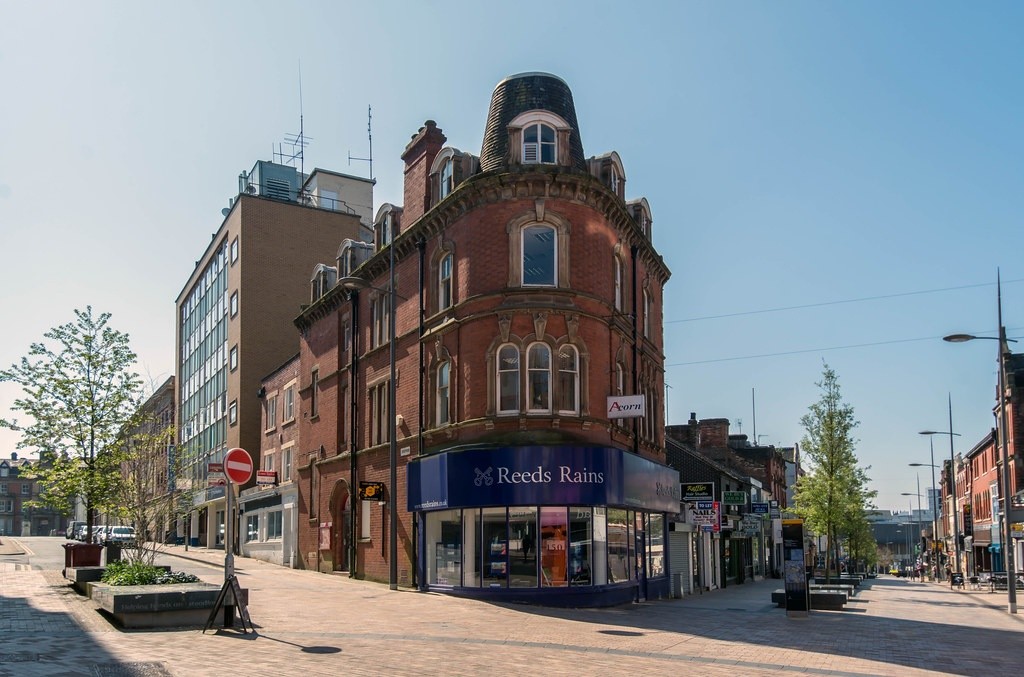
[889,560,907,577]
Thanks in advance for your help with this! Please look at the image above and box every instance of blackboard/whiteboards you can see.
[951,573,965,585]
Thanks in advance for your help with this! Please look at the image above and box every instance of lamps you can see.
[256,386,266,397]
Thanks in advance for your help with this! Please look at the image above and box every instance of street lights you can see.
[337,276,398,589]
[942,333,1018,614]
[909,463,941,583]
[920,430,961,588]
[901,493,924,583]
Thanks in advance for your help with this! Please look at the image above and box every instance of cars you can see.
[77,526,138,548]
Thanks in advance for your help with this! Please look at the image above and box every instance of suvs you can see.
[66,521,87,539]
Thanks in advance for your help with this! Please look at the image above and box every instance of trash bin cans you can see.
[106,541,121,564]
[1,529,4,535]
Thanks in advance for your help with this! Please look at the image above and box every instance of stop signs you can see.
[222,448,253,485]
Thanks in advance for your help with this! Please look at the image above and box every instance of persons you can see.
[933,565,940,572]
[944,559,953,581]
